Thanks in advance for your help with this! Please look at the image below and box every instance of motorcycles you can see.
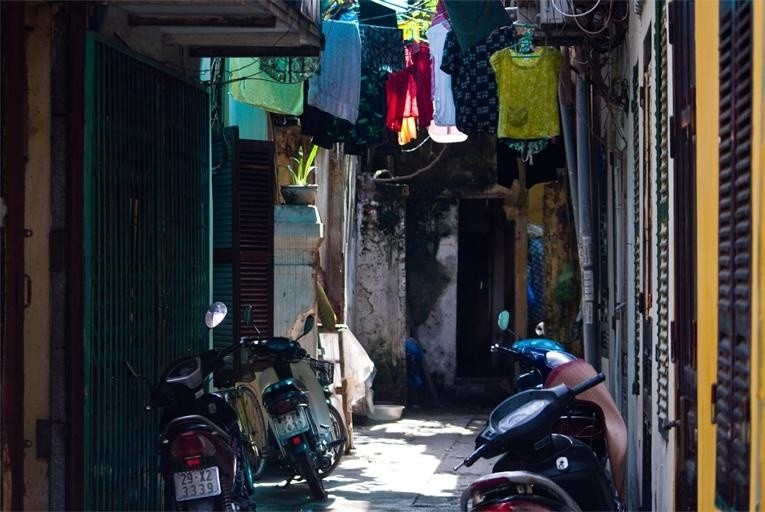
[489,312,569,387]
[243,304,347,496]
[123,301,258,511]
[452,373,628,512]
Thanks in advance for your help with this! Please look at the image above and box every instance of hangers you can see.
[509,22,543,59]
[406,28,417,44]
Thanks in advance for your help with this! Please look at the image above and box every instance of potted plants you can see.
[276,145,318,205]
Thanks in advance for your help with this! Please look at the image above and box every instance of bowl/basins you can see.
[367,404,405,421]
